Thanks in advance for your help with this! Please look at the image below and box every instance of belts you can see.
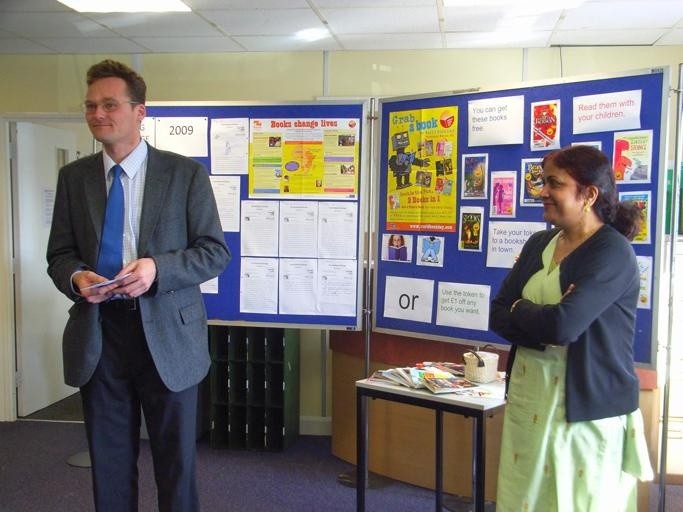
[95,296,141,314]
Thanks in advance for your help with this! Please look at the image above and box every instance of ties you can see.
[92,164,128,306]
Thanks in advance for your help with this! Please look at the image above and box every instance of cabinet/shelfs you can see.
[194,325,299,451]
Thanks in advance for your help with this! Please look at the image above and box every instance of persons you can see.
[488,146,655,512]
[388,235,404,247]
[46,58,232,512]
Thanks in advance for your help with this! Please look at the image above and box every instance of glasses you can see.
[81,99,143,113]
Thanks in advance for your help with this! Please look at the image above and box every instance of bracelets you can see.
[510,299,523,312]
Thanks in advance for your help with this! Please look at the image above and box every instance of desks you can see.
[353,368,509,511]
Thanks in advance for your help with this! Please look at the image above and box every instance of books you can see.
[388,246,407,261]
[367,361,480,394]
